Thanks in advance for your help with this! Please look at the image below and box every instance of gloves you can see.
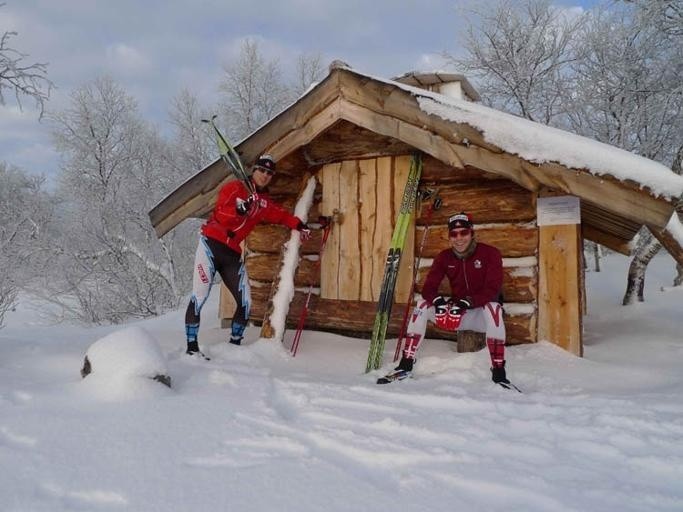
[241,192,259,212]
[432,296,448,330]
[297,222,312,242]
[447,299,471,330]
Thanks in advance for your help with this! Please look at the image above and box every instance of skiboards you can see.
[203,113,257,200]
[365,156,422,368]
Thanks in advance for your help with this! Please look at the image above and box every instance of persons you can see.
[377,211,521,393]
[185,154,312,361]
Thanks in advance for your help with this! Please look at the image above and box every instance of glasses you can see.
[449,230,469,238]
[256,168,272,175]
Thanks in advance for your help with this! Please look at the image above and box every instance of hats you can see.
[448,211,473,231]
[254,155,276,171]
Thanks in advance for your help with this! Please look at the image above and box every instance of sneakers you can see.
[386,367,410,378]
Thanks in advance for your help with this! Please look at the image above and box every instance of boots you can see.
[186,339,210,361]
[229,337,244,345]
